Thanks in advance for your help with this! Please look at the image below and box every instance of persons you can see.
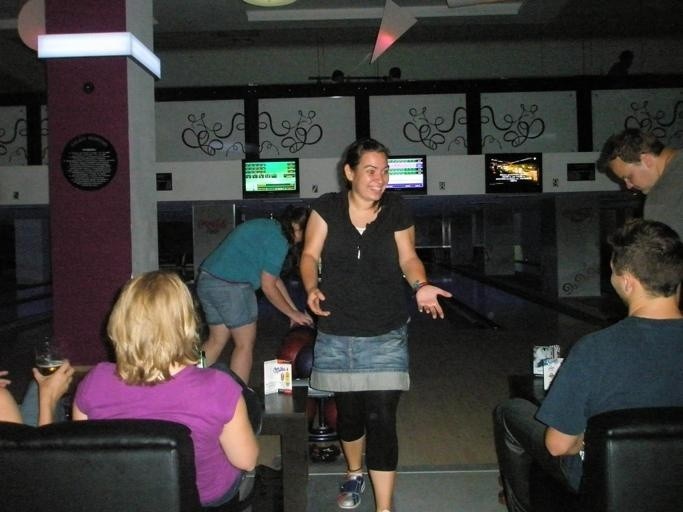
[596,126,683,244]
[71,269,261,507]
[299,136,453,512]
[489,218,682,511]
[0,357,77,427]
[196,203,313,385]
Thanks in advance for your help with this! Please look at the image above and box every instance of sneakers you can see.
[337,475,365,509]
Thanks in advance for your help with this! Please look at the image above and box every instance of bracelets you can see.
[414,281,430,294]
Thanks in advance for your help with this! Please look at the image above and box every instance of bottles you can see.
[196,350,207,368]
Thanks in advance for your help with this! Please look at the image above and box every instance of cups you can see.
[36,339,69,375]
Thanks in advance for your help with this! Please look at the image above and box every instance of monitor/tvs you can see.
[242,158,300,199]
[384,155,427,195]
[485,153,542,194]
